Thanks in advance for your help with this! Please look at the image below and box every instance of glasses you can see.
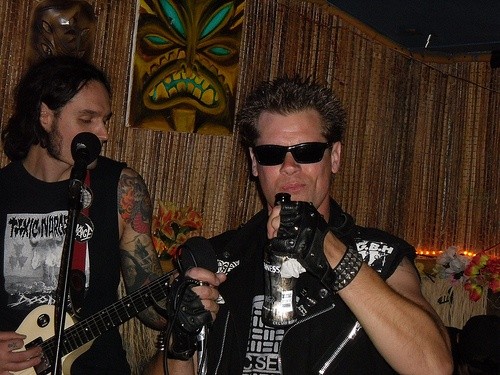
[251,141,333,166]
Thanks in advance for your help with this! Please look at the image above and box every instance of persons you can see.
[0,53,169,375]
[146,74,455,375]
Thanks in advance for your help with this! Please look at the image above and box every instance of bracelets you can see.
[332,244,362,292]
[159,336,194,361]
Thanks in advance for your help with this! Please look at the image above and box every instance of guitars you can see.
[9,268,181,375]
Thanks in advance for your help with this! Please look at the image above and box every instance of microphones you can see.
[67,131,102,199]
[182,236,218,374]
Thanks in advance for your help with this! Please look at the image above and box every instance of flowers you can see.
[151,196,204,261]
[432,245,500,303]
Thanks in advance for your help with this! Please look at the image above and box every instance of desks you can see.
[414,254,489,348]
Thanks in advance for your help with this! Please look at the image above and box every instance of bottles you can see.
[261,194,298,327]
